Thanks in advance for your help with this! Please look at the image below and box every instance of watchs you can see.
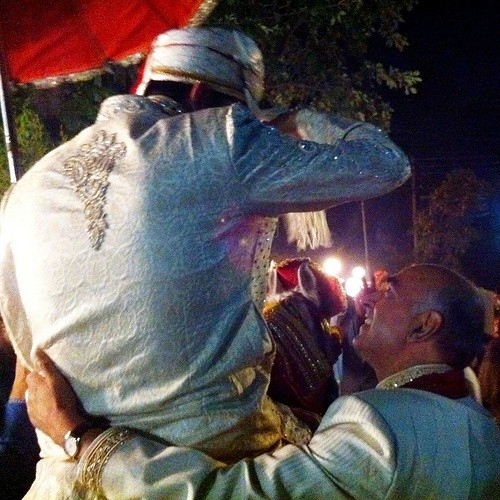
[62,413,113,462]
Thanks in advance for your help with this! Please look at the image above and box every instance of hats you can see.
[138,28,266,108]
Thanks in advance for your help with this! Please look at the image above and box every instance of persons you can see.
[332,286,381,399]
[0,26,410,466]
[21,261,500,500]
